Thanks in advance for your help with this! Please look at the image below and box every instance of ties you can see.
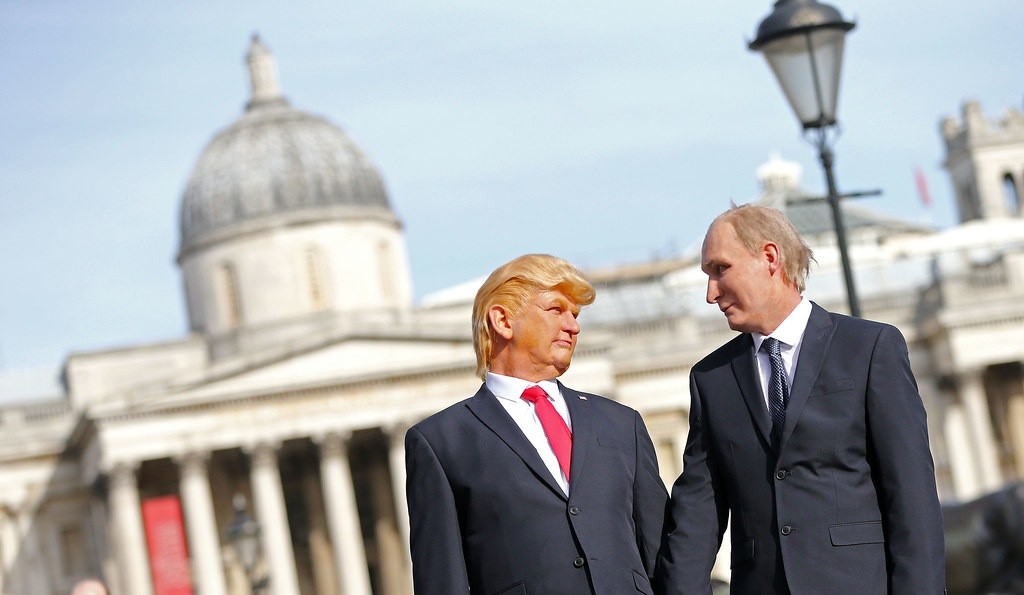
[761,338,789,443]
[522,386,573,480]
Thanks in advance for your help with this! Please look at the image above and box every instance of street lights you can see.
[744,0,864,319]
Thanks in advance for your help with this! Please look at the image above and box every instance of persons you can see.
[404,256,714,595]
[652,204,947,594]
